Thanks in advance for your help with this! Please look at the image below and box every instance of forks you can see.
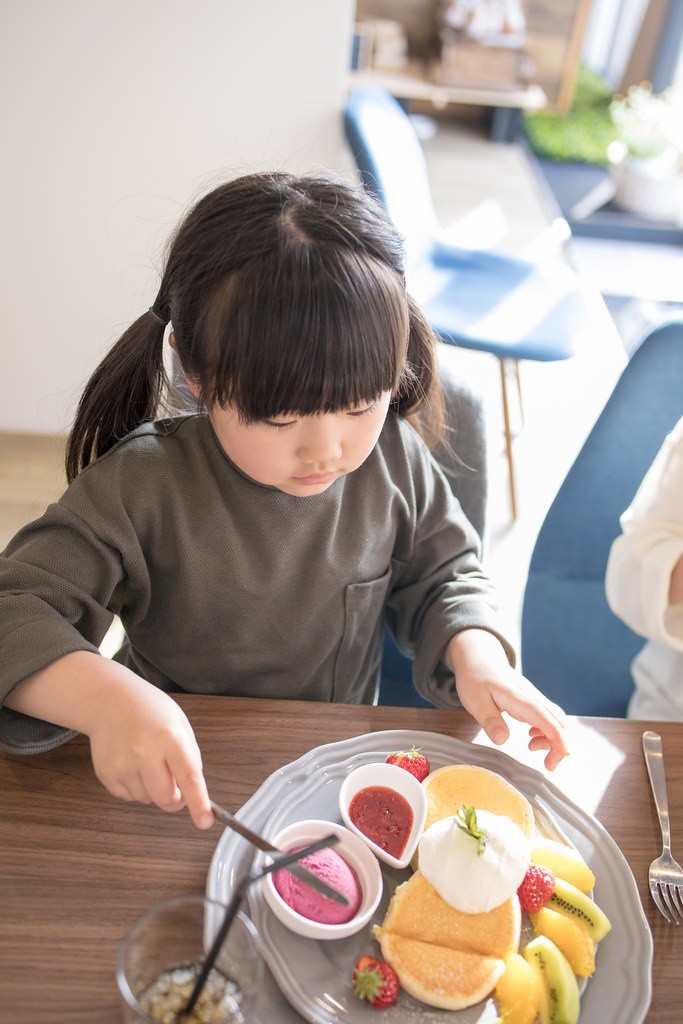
[640,729,683,925]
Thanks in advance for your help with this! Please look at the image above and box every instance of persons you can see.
[0,172,577,829]
[604,423,683,724]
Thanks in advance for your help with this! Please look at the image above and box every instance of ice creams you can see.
[273,843,363,924]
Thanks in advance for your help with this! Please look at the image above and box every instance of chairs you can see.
[163,342,489,545]
[517,320,683,723]
[343,80,583,522]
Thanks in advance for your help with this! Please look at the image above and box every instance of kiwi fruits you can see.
[524,936,580,1024]
[543,877,611,943]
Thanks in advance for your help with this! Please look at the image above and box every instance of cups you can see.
[113,896,264,1024]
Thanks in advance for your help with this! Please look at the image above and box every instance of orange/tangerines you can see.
[529,907,597,979]
[491,956,541,1024]
[530,835,595,893]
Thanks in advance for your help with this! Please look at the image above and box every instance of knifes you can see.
[208,799,350,907]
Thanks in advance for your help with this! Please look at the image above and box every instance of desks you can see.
[360,54,550,144]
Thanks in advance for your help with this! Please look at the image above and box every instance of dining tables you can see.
[0,685,683,1024]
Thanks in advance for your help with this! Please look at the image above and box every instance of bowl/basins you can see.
[337,761,428,871]
[263,818,385,942]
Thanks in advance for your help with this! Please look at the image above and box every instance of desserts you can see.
[407,763,535,875]
[372,869,522,1009]
[416,807,532,916]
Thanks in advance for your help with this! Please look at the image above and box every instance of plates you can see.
[203,730,655,1024]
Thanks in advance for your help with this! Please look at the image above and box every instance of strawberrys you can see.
[385,744,430,783]
[353,956,398,1008]
[517,863,555,914]
